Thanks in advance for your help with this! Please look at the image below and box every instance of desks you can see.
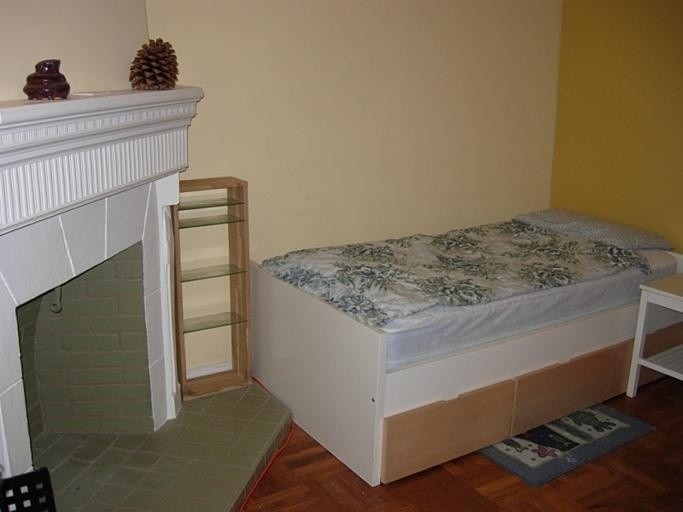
[626,273,683,398]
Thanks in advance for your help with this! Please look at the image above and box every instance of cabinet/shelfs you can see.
[172,176,251,402]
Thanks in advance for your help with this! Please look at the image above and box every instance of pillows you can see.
[514,211,674,250]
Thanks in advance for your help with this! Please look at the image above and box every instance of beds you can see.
[246,210,683,488]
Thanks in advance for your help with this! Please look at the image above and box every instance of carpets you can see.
[479,401,655,488]
